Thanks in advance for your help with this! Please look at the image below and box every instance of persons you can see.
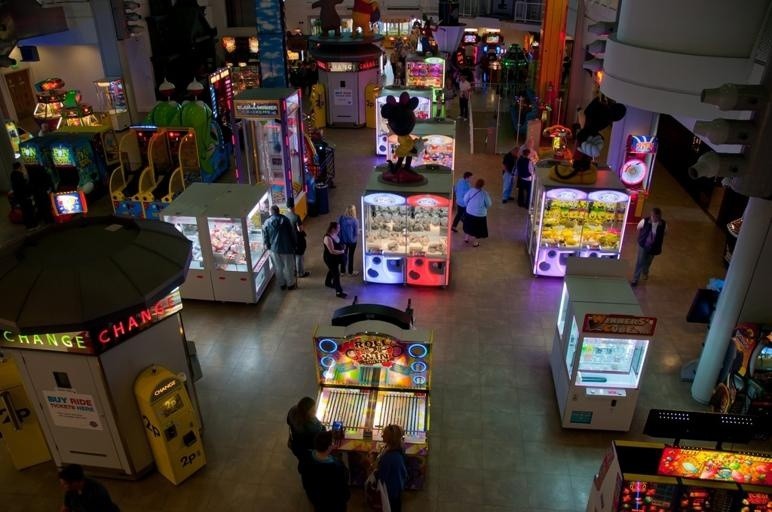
[629,208,669,288]
[336,205,360,278]
[452,172,474,232]
[456,74,472,121]
[286,396,409,512]
[515,148,535,209]
[59,464,121,512]
[287,60,318,96]
[283,198,310,279]
[264,206,299,291]
[453,64,460,90]
[323,221,348,299]
[489,115,500,127]
[502,146,520,204]
[9,160,40,229]
[463,178,492,246]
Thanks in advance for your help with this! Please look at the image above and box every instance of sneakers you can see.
[280,284,295,291]
[297,272,309,278]
[340,270,360,277]
[452,227,481,247]
[325,282,347,298]
[502,197,515,204]
[630,272,648,289]
